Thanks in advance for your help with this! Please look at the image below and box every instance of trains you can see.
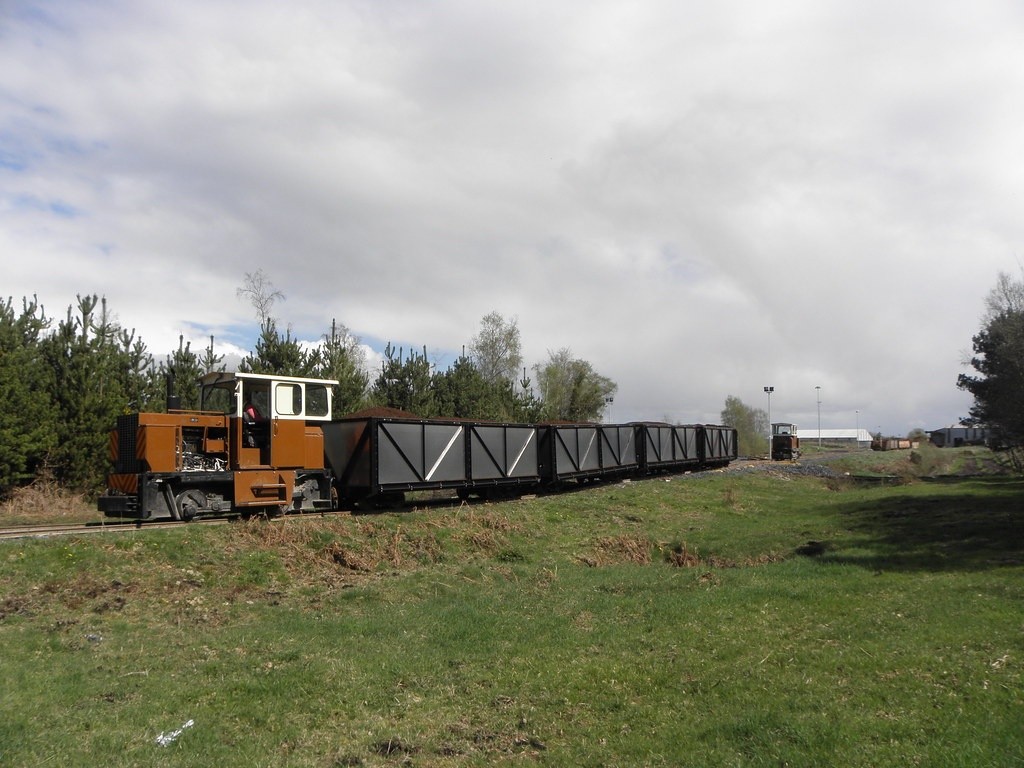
[871,434,919,451]
[86,363,738,526]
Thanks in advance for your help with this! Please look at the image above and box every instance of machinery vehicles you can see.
[771,423,801,461]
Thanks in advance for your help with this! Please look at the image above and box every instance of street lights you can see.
[815,386,822,449]
[763,386,776,460]
